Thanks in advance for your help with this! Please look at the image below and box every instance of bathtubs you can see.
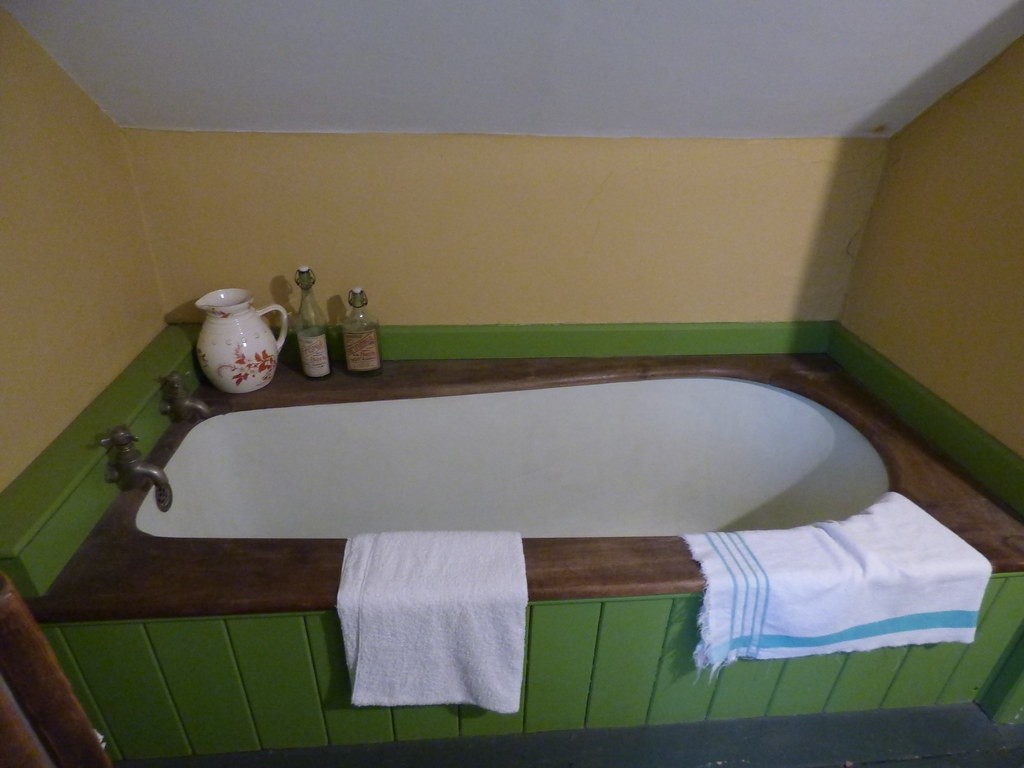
[137,377,894,538]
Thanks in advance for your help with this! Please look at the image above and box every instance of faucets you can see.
[98,427,173,512]
[159,373,211,422]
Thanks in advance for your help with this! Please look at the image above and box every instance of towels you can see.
[680,491,993,686]
[337,531,528,713]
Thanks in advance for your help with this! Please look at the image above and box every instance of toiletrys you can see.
[340,288,381,375]
[293,268,333,378]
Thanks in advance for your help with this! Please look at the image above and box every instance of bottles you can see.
[342,287,383,374]
[293,265,331,378]
